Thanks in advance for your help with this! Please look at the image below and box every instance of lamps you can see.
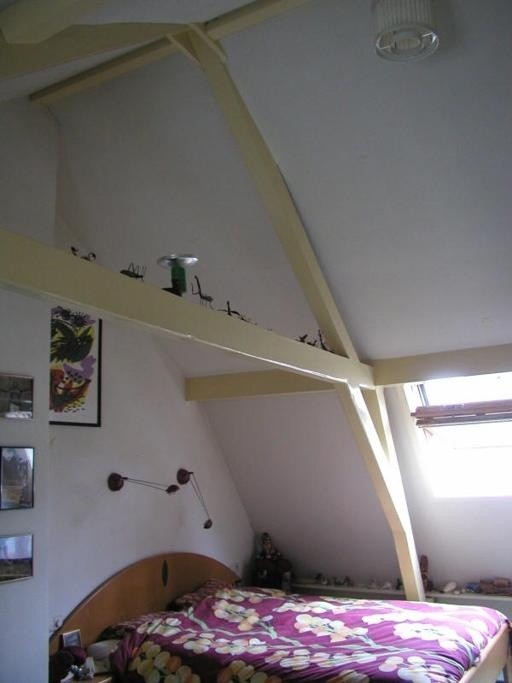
[371,0,442,63]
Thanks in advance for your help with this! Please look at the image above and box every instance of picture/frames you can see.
[1,371,37,587]
[63,627,82,647]
[50,303,102,425]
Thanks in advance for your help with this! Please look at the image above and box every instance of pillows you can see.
[103,577,233,636]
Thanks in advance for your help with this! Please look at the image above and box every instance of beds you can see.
[50,552,512,683]
